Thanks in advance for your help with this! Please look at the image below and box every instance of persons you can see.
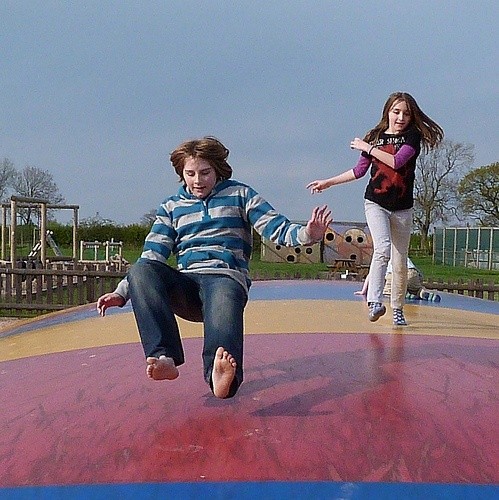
[354,242,441,302]
[307,92,444,326]
[95,135,332,400]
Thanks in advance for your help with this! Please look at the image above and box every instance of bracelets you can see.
[367,145,374,155]
